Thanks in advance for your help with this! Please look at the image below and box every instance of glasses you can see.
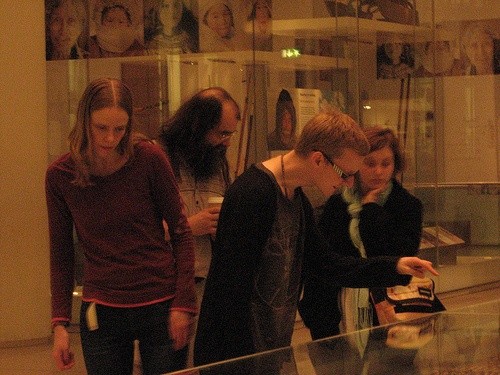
[311,149,361,182]
[213,126,240,139]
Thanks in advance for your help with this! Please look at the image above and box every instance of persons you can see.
[379,22,500,75]
[44,77,439,375]
[268,89,299,150]
[45,0,271,60]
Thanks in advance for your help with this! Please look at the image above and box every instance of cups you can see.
[207,196,224,208]
